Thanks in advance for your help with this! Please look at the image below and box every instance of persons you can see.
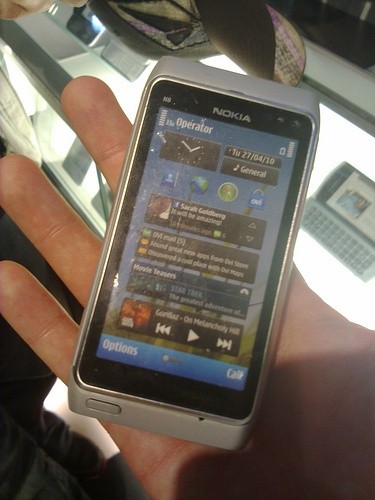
[0,76,374,500]
[0,0,115,500]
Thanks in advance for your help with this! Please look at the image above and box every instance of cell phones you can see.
[68,56,320,452]
[301,162,375,282]
